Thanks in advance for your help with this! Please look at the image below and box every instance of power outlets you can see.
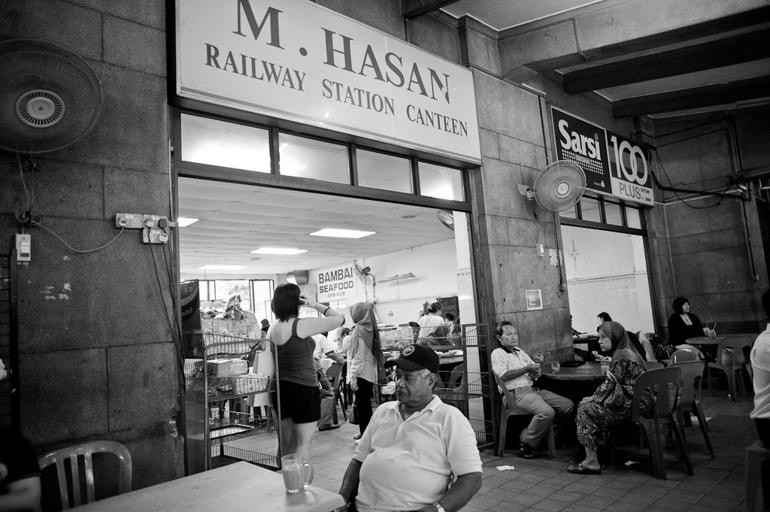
[115,212,169,245]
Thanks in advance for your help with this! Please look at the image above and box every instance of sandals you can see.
[518,431,534,459]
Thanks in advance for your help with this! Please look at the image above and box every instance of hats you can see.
[383,343,440,375]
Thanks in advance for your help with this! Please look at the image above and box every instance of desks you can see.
[65,459,346,512]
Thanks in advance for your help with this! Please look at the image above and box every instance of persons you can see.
[667,296,710,371]
[570,299,663,475]
[749,314,770,452]
[491,321,574,459]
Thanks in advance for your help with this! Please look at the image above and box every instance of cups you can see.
[280,454,314,494]
[551,361,560,373]
[601,359,610,375]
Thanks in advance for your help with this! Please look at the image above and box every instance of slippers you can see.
[567,462,602,475]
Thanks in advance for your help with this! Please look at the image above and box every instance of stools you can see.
[744,442,768,512]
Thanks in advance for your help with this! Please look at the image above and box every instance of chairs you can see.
[181,329,760,478]
[37,440,134,512]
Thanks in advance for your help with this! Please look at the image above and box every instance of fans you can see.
[437,208,454,232]
[351,261,376,286]
[525,159,589,214]
[1,37,104,174]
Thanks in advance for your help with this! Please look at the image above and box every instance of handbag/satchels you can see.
[349,398,359,425]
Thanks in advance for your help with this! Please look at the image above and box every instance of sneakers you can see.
[319,423,341,432]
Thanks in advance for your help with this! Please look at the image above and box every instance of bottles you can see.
[381,322,416,352]
[703,328,717,341]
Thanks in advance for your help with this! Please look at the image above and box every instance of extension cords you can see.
[115,213,169,230]
